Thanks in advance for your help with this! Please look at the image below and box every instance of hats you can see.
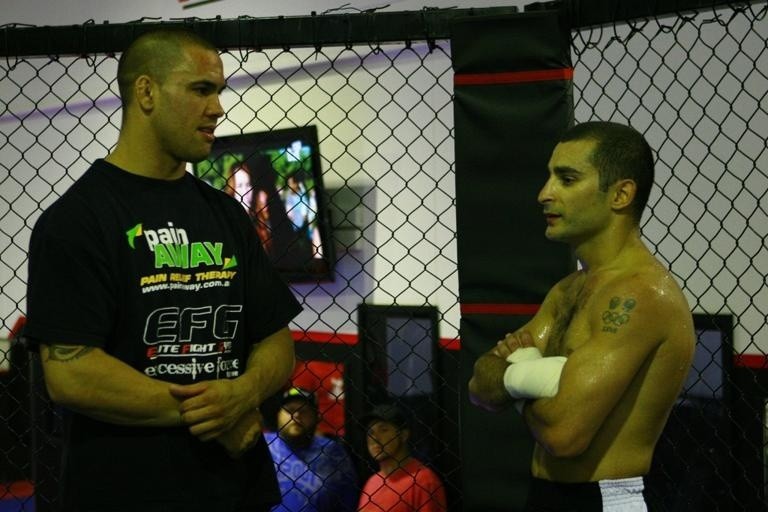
[364,404,408,426]
[280,387,314,403]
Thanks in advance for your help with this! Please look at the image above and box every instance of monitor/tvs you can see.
[192,125,336,284]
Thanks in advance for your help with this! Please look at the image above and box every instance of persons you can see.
[27,31,304,512]
[284,173,309,231]
[359,408,446,512]
[224,160,252,217]
[253,184,287,261]
[264,388,352,512]
[470,121,694,512]
